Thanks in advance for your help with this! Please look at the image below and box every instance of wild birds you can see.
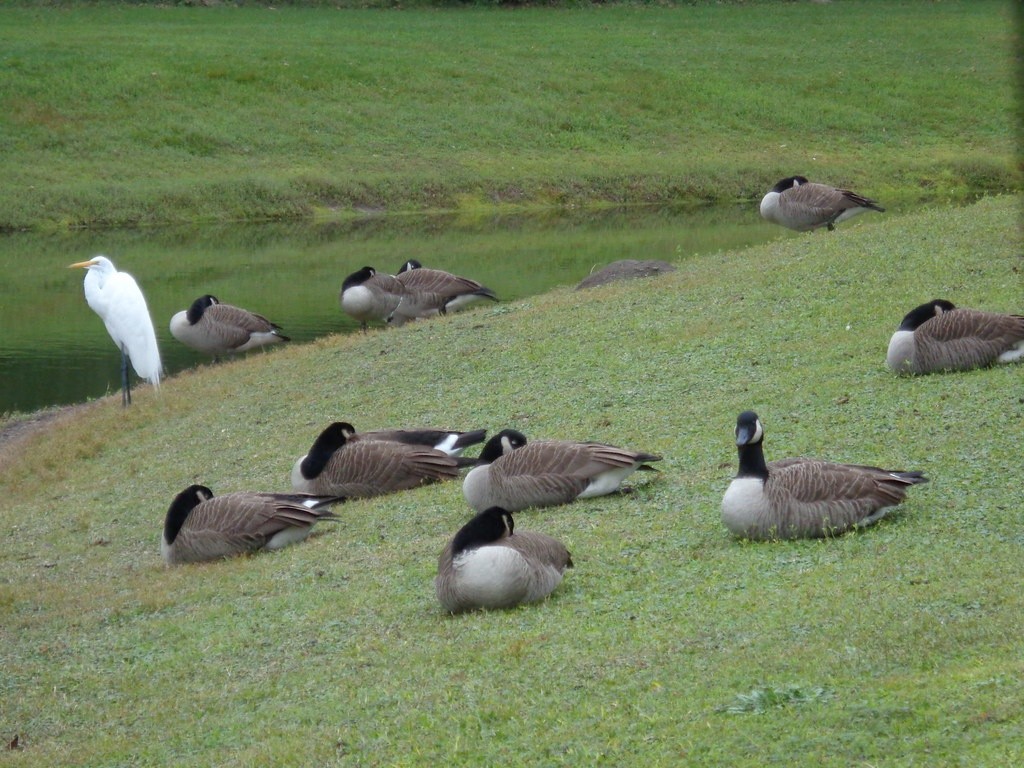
[64,176,1024,613]
